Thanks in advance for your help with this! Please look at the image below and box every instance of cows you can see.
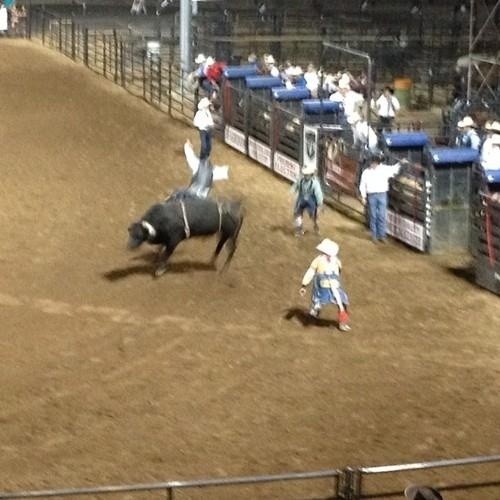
[127,198,244,277]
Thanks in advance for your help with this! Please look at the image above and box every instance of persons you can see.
[298,237,353,332]
[187,52,499,172]
[359,155,409,246]
[287,164,324,237]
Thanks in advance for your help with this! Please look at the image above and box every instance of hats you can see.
[197,97,211,109]
[316,238,339,257]
[300,164,315,174]
[457,117,480,129]
[484,119,500,132]
